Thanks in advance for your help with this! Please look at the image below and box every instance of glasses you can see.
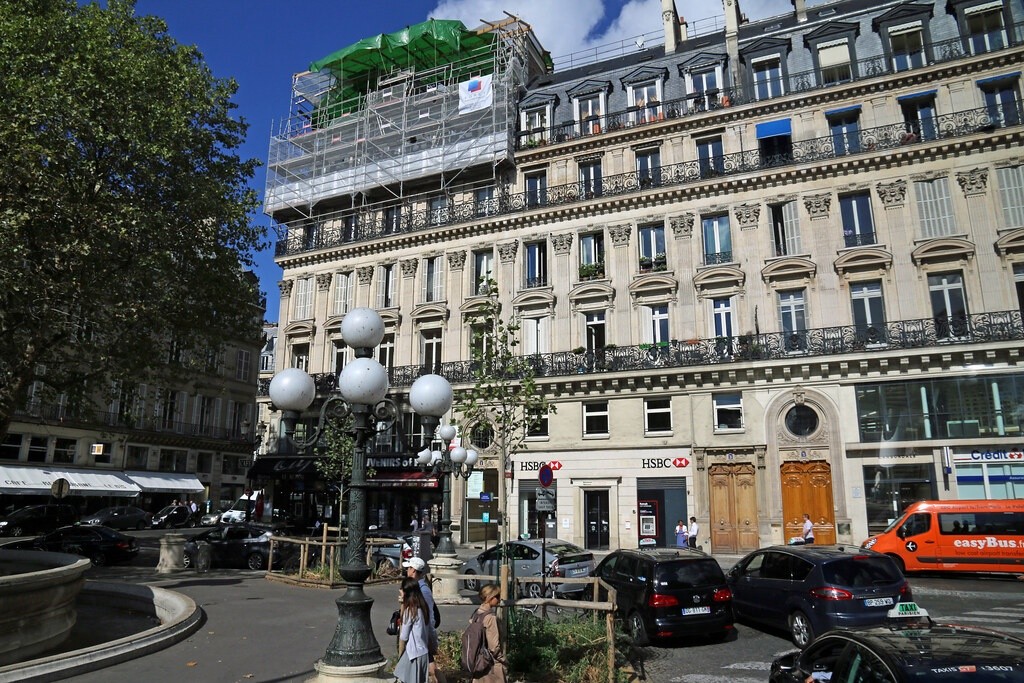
[405,566,412,570]
[493,594,500,600]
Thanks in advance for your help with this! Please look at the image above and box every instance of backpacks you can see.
[461,609,492,674]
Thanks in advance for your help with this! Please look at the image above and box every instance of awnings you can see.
[0,465,142,498]
[123,470,205,493]
[247,459,316,481]
[366,469,441,487]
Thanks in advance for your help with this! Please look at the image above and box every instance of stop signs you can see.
[245,489,251,495]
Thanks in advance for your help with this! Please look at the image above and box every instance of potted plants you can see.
[522,139,537,150]
[654,253,666,265]
[671,337,678,346]
[579,262,604,280]
[639,255,652,269]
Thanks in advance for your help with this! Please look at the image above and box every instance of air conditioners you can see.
[90,444,103,455]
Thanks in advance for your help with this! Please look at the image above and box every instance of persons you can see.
[685,517,699,548]
[392,577,432,683]
[402,557,447,683]
[804,671,833,683]
[465,583,511,683]
[802,514,815,543]
[172,500,197,522]
[673,519,688,546]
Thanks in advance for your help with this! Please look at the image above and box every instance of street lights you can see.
[240,418,267,523]
[268,307,454,683]
[415,426,478,600]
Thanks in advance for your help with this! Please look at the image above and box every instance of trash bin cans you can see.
[427,557,464,605]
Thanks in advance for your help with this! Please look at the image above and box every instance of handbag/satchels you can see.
[433,604,441,628]
[386,610,400,635]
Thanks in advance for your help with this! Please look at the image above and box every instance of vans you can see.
[859,498,1024,577]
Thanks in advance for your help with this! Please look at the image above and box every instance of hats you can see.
[402,557,425,572]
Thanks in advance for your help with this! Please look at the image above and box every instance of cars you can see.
[80,505,154,530]
[725,537,912,649]
[0,505,81,537]
[768,601,1024,683]
[201,490,285,526]
[183,525,319,571]
[18,524,140,567]
[152,505,196,529]
[459,538,597,598]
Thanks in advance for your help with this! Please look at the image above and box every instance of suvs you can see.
[582,545,735,645]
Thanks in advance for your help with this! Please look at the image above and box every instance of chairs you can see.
[952,521,962,534]
[959,521,970,533]
[515,547,528,559]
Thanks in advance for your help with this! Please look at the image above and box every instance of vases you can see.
[539,139,545,148]
